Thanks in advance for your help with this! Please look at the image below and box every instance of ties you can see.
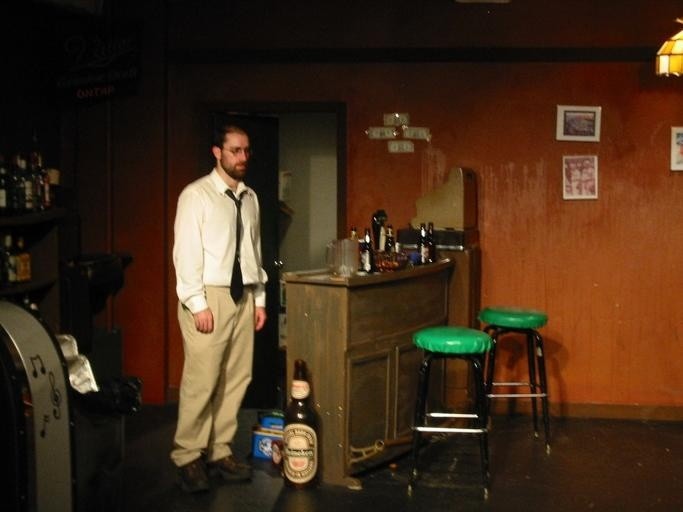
[226,188,247,306]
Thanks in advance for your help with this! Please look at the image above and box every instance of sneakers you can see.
[206,452,255,483]
[172,458,212,494]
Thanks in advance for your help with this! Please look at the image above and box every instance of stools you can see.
[478,306,552,454]
[407,326,495,499]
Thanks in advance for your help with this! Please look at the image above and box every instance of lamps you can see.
[655,17,683,77]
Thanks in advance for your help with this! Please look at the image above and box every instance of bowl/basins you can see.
[376,252,408,269]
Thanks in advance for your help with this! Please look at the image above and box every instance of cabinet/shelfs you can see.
[1,211,141,481]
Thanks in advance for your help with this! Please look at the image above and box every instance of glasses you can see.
[222,146,253,156]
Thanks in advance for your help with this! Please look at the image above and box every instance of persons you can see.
[564,159,594,198]
[171,120,267,498]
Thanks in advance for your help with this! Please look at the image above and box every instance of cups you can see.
[323,238,360,283]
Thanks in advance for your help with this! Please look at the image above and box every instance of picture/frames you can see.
[555,104,602,143]
[670,125,683,171]
[562,154,599,200]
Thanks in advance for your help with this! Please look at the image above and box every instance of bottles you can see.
[372,210,387,253]
[362,228,373,274]
[349,225,358,239]
[0,136,52,216]
[419,222,438,262]
[385,225,401,253]
[0,233,35,285]
[283,357,323,488]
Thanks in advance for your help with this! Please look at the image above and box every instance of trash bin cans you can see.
[19,394,128,506]
